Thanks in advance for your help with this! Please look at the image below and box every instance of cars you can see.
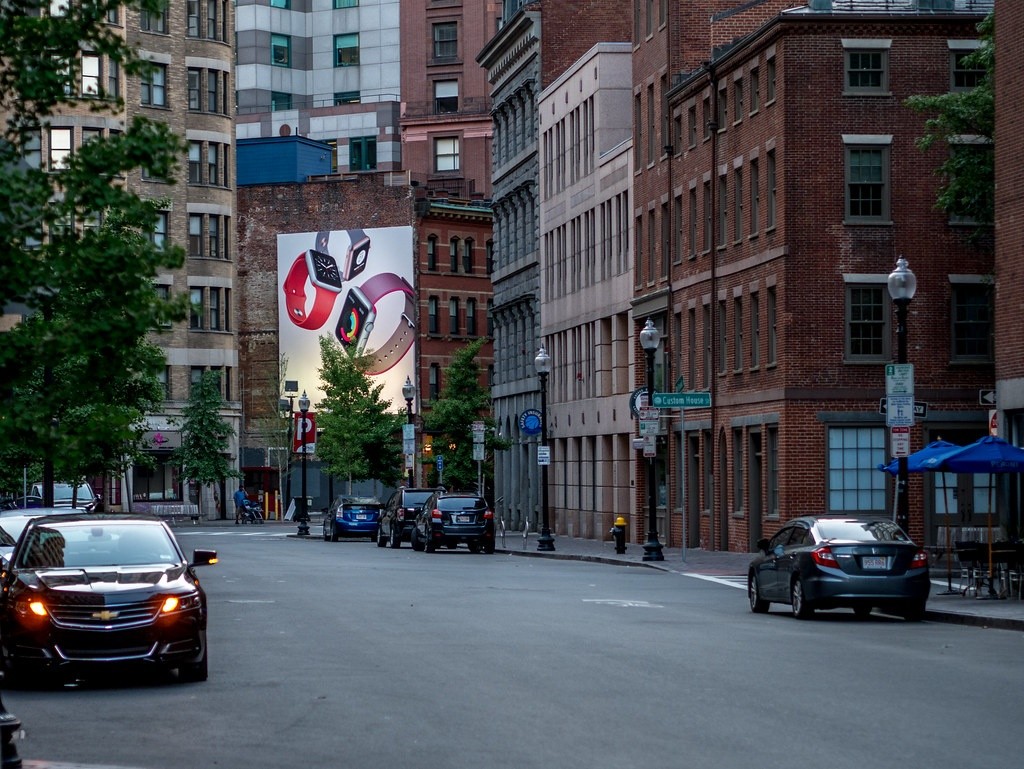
[0,508,113,595]
[0,512,219,685]
[747,512,930,623]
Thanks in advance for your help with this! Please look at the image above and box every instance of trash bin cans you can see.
[292,495,313,522]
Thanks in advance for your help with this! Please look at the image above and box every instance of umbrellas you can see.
[877,441,965,589]
[918,435,1024,591]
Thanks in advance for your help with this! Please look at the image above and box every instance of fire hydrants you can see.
[610,515,628,554]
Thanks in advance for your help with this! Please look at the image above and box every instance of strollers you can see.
[240,499,266,525]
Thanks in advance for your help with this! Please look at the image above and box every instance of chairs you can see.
[118,531,158,561]
[956,540,1024,602]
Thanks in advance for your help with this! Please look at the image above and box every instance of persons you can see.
[188,488,200,525]
[214,490,220,512]
[233,485,249,524]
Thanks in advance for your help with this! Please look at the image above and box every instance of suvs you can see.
[29,479,103,516]
[320,494,382,543]
[411,491,496,554]
[376,484,451,549]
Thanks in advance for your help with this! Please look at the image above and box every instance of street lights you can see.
[297,389,311,537]
[887,251,917,536]
[535,342,556,551]
[640,310,670,559]
[401,375,416,488]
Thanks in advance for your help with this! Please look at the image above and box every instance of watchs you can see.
[282,248,343,330]
[335,271,418,377]
[315,229,372,282]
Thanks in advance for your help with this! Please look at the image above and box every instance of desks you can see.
[923,546,962,595]
